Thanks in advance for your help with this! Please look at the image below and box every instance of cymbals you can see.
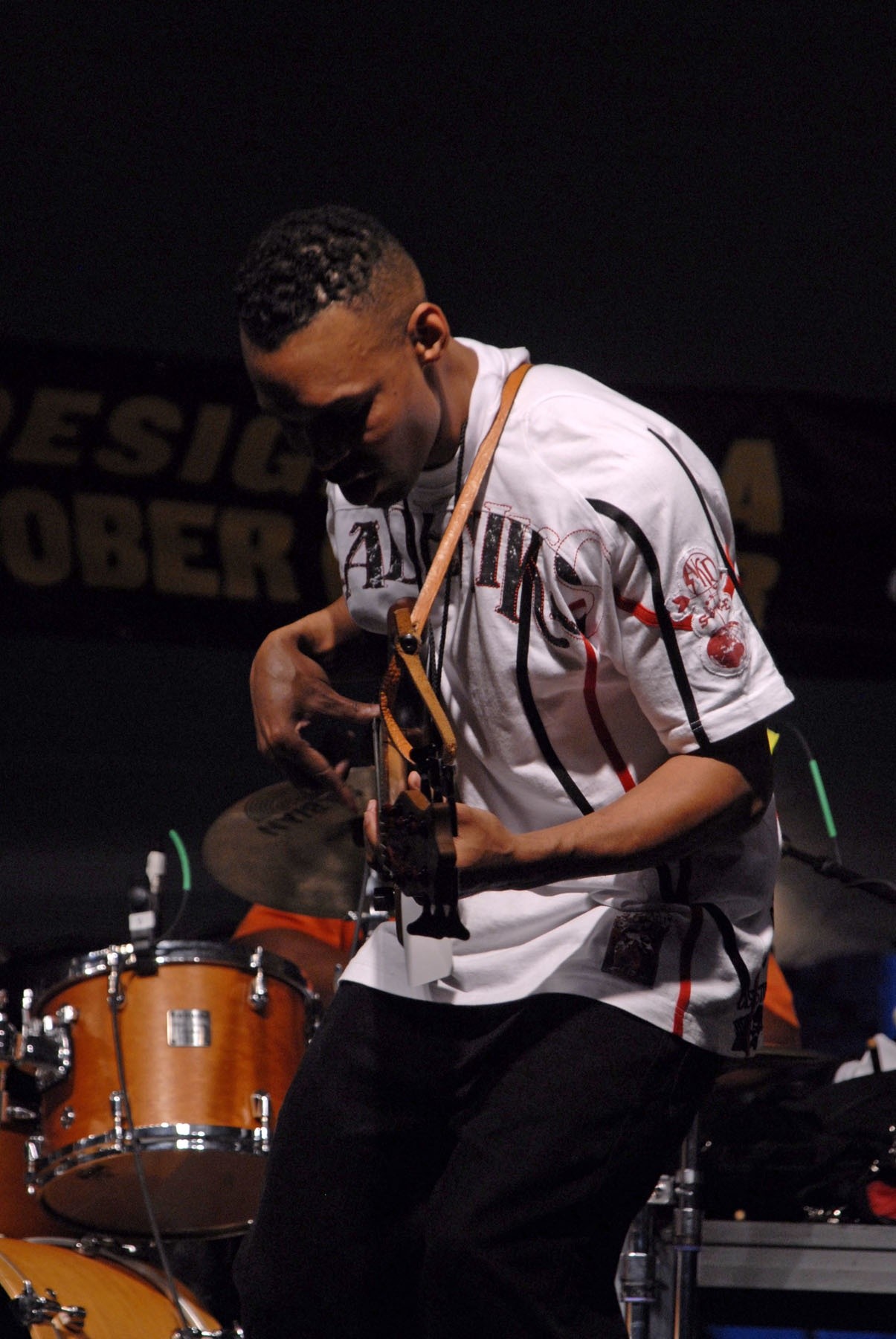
[199,767,395,918]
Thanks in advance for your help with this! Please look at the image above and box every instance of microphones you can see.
[142,835,172,915]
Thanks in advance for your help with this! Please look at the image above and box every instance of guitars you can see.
[373,596,476,944]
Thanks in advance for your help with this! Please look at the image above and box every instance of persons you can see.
[228,206,802,1339]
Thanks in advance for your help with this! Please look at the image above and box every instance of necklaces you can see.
[401,414,467,692]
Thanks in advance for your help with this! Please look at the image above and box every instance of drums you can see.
[19,938,320,1237]
[0,1231,236,1339]
